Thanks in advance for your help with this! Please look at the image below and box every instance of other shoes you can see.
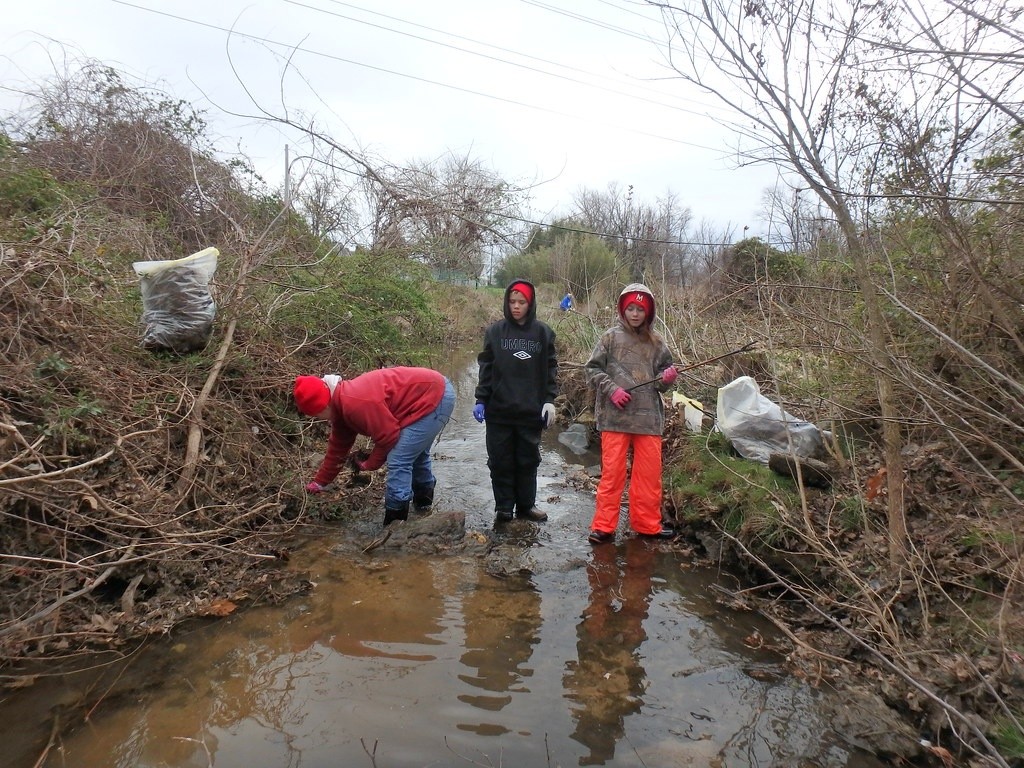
[589,530,612,543]
[640,527,675,539]
[496,506,548,521]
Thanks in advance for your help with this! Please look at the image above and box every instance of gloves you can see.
[663,366,678,385]
[306,482,322,492]
[611,386,633,411]
[474,405,485,422]
[541,403,556,429]
[354,457,368,471]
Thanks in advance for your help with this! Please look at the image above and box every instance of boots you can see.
[384,509,408,529]
[412,490,433,510]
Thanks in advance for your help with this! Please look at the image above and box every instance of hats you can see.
[295,376,332,416]
[621,292,651,318]
[511,283,533,304]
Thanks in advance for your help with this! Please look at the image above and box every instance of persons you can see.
[294,365,456,529]
[582,281,683,544]
[560,292,575,314]
[471,278,562,525]
